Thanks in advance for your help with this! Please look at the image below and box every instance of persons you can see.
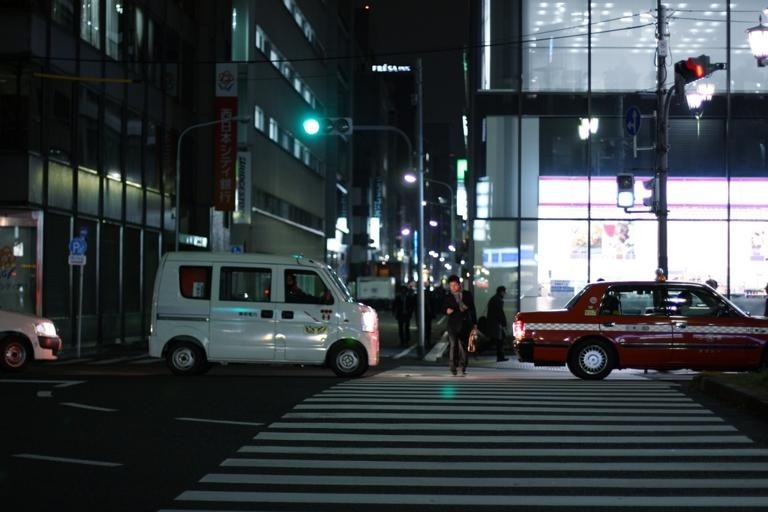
[676,291,717,316]
[288,275,305,295]
[442,275,477,375]
[391,278,448,349]
[472,285,510,362]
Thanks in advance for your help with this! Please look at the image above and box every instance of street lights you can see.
[577,113,598,178]
[685,81,720,139]
[174,115,252,250]
[400,173,457,275]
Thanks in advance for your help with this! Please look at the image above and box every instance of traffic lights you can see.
[675,55,707,82]
[617,173,634,208]
[301,114,354,136]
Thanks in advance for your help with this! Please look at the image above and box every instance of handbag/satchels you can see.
[466,327,483,354]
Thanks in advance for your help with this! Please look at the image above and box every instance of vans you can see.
[145,250,382,377]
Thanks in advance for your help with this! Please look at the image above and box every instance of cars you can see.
[0,308,63,370]
[511,268,768,381]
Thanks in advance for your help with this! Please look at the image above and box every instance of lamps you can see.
[686,92,703,113]
[697,83,715,105]
